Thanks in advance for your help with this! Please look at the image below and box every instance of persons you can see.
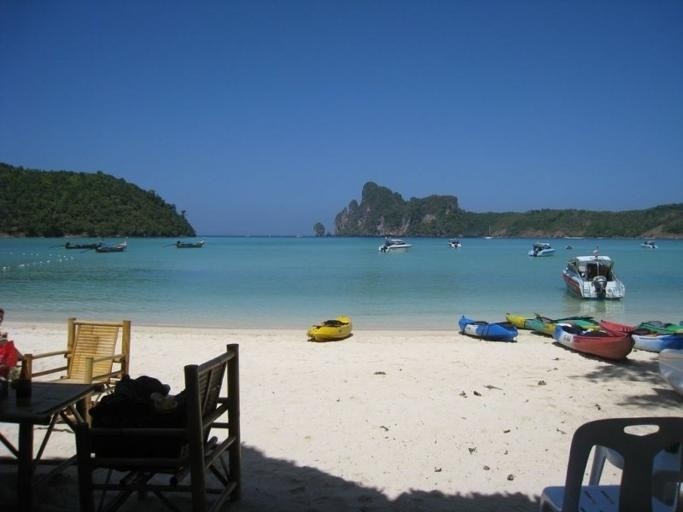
[0,308,10,381]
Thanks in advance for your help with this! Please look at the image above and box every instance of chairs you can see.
[72,343,241,511]
[534,416,682,512]
[19,314,133,427]
[587,436,682,512]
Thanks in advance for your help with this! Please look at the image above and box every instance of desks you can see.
[0,379,105,511]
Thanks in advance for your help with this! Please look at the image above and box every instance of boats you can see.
[505,312,602,337]
[552,323,635,361]
[458,315,518,339]
[175,240,205,248]
[65,242,106,249]
[561,254,626,301]
[307,316,352,342]
[601,320,683,353]
[526,241,554,256]
[636,319,683,333]
[378,238,413,253]
[641,241,659,250]
[448,240,463,250]
[95,238,129,253]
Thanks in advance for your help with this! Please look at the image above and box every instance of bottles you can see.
[0,352,10,396]
[17,358,33,408]
